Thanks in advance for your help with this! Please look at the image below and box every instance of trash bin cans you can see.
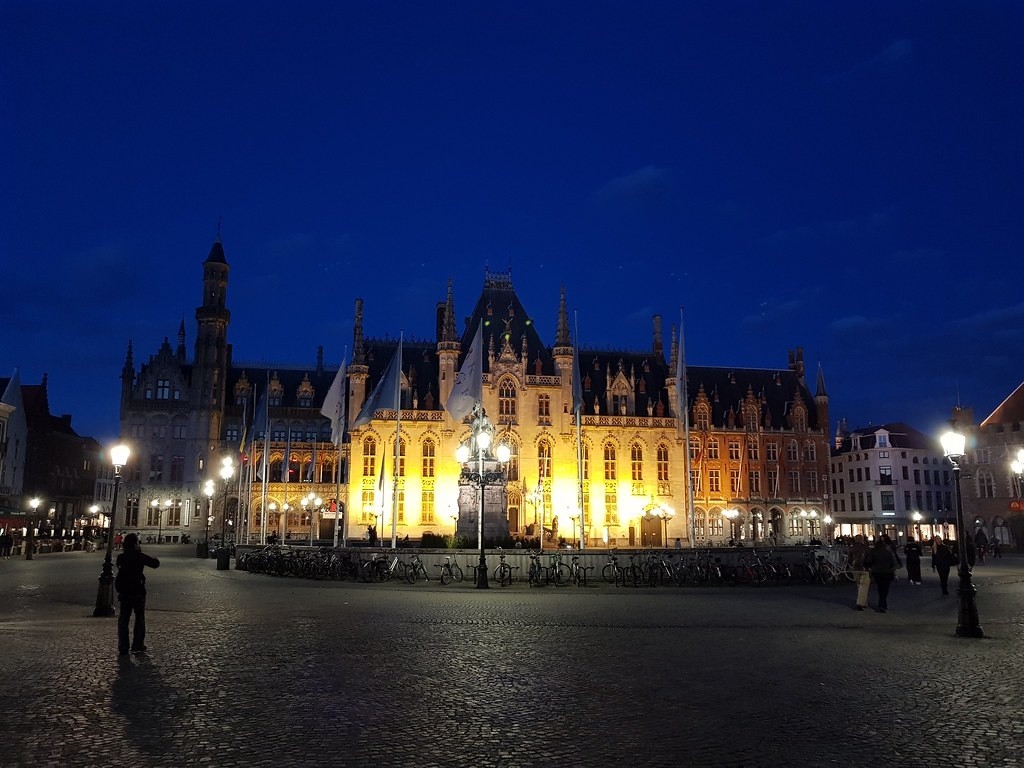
[196,542,209,558]
[217,547,230,570]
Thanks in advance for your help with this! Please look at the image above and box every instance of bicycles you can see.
[601,546,623,587]
[548,548,572,587]
[491,545,512,588]
[525,549,546,588]
[404,550,430,584]
[641,549,861,588]
[439,553,464,586]
[228,540,407,584]
[626,553,645,587]
[566,549,585,587]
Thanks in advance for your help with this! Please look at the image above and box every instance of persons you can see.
[286,531,291,539]
[115,533,160,652]
[0,532,13,559]
[836,534,923,614]
[770,531,776,545]
[267,530,278,545]
[114,531,142,550]
[931,535,953,598]
[675,538,682,547]
[471,407,494,459]
[368,525,377,547]
[965,529,1002,567]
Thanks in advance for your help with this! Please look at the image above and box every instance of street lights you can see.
[91,442,132,620]
[270,503,289,542]
[24,497,40,560]
[149,497,175,545]
[823,515,833,548]
[453,401,513,590]
[202,479,216,541]
[938,430,987,638]
[912,511,924,556]
[89,505,111,540]
[652,502,675,548]
[721,509,739,539]
[364,498,382,528]
[218,456,234,547]
[801,509,817,541]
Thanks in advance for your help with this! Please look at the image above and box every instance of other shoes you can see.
[916,581,922,585]
[118,647,130,654]
[132,644,147,651]
[909,579,913,584]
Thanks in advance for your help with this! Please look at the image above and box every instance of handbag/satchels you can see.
[949,553,960,566]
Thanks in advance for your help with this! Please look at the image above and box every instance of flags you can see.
[244,385,267,456]
[352,339,402,426]
[237,430,349,484]
[677,312,687,417]
[571,312,582,428]
[321,356,345,445]
[446,318,482,420]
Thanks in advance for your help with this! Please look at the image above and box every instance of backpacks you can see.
[114,553,146,595]
